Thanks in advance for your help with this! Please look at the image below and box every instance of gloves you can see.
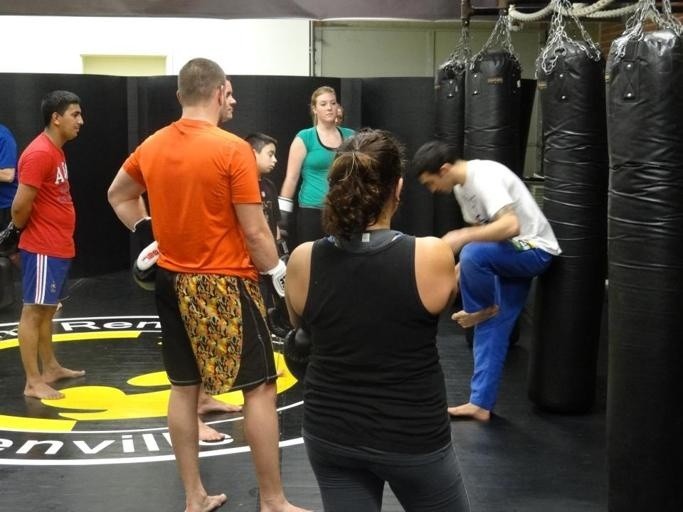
[276,196,295,235]
[130,216,158,292]
[283,328,312,383]
[258,258,293,334]
[0,222,23,258]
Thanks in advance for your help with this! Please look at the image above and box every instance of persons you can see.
[277,85,365,245]
[2,90,85,400]
[410,139,561,425]
[106,55,308,512]
[331,101,345,128]
[282,128,469,512]
[243,126,295,348]
[170,73,243,444]
[1,123,63,311]
